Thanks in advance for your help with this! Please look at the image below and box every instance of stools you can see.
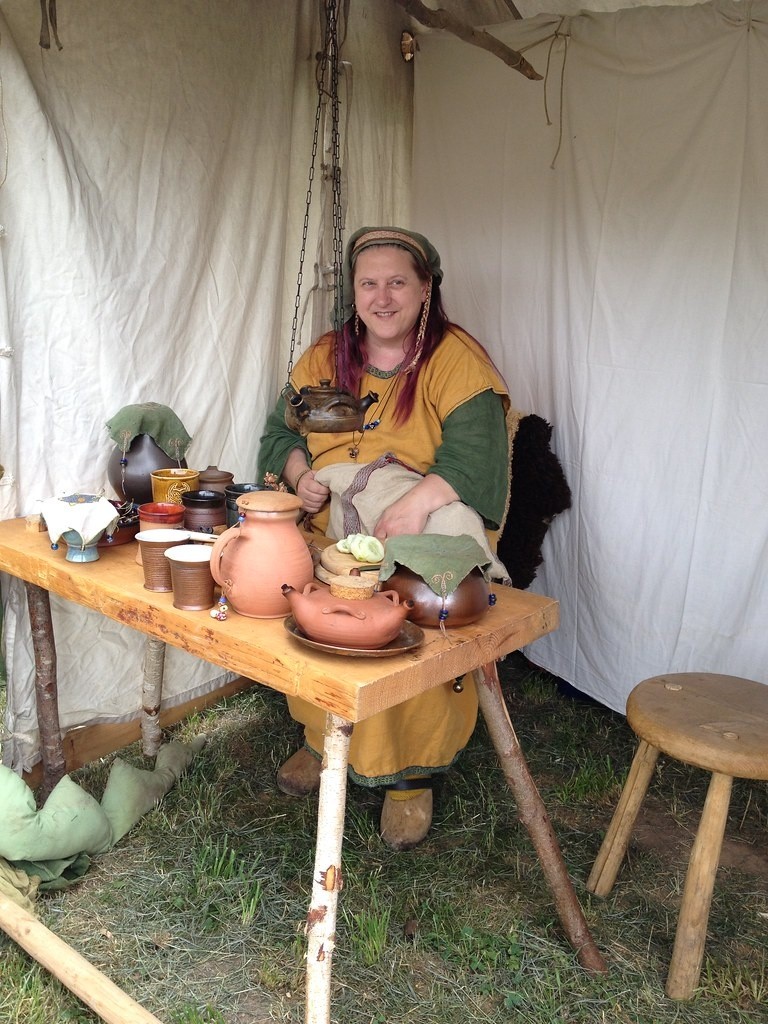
[585,672,768,1001]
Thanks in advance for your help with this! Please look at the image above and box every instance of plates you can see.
[282,614,425,657]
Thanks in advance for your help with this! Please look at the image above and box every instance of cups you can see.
[150,468,200,505]
[224,483,275,528]
[197,466,234,494]
[181,490,226,534]
[135,503,187,567]
[135,528,190,593]
[163,544,213,611]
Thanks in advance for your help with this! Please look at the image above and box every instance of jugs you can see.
[280,568,415,650]
[381,565,497,628]
[210,491,314,618]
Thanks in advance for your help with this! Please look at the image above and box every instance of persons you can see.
[258,225,511,849]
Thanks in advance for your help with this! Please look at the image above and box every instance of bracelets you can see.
[295,470,310,493]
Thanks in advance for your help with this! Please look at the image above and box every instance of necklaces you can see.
[349,349,410,459]
[362,371,402,431]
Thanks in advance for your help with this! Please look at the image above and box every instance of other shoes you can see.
[379,773,434,850]
[276,747,322,797]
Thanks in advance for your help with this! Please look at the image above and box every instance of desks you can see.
[0,515,604,1024]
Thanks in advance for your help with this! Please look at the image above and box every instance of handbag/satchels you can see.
[305,452,513,587]
